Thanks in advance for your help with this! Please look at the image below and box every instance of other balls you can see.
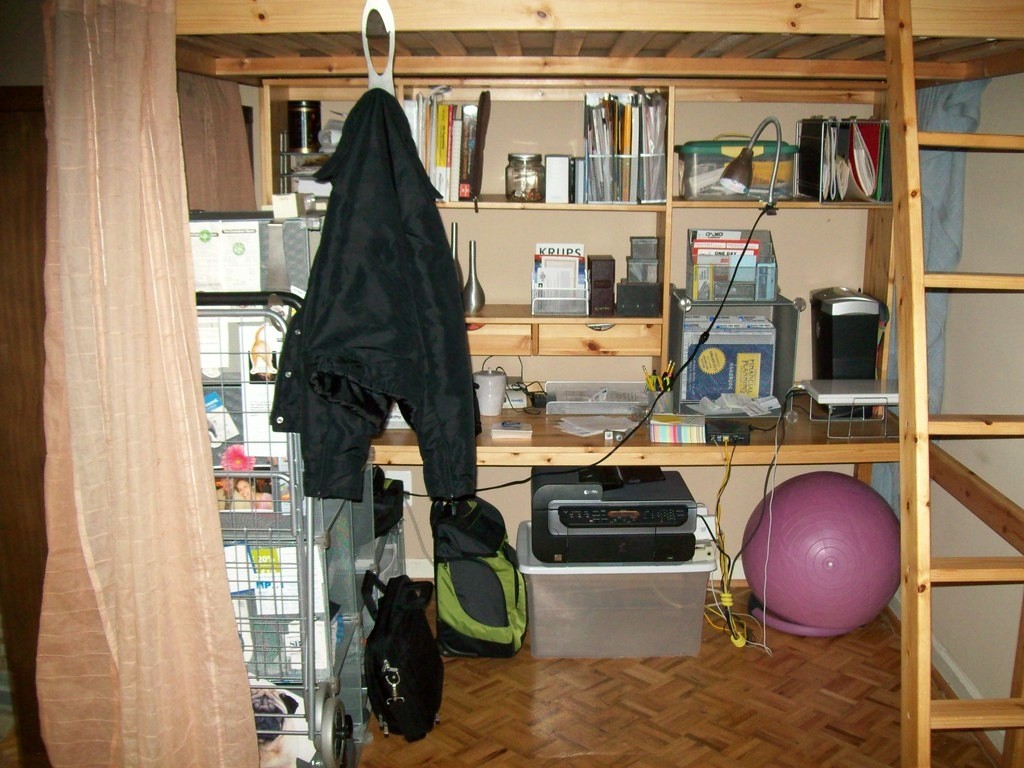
[738,469,908,631]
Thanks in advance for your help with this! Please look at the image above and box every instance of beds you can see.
[173,0,1024,113]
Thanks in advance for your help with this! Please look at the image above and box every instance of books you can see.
[403,91,668,205]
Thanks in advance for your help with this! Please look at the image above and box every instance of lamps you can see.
[719,115,784,217]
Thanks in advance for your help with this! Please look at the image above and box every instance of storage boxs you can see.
[639,153,666,206]
[587,253,616,317]
[189,213,273,292]
[625,255,659,283]
[516,520,717,659]
[613,155,639,205]
[617,278,659,319]
[630,236,659,259]
[587,153,612,205]
[681,137,801,201]
[669,285,806,426]
[544,153,575,204]
[684,226,782,303]
[298,177,332,197]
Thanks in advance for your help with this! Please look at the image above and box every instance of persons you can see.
[216,476,272,510]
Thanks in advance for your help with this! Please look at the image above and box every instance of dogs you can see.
[248,685,317,768]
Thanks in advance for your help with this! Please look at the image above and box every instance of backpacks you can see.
[430,496,528,658]
[372,464,403,538]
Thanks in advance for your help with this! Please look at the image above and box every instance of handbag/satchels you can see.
[360,570,444,743]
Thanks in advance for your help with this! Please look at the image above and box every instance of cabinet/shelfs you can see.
[258,76,929,463]
[197,292,378,768]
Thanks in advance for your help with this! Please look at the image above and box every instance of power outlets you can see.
[384,470,413,508]
